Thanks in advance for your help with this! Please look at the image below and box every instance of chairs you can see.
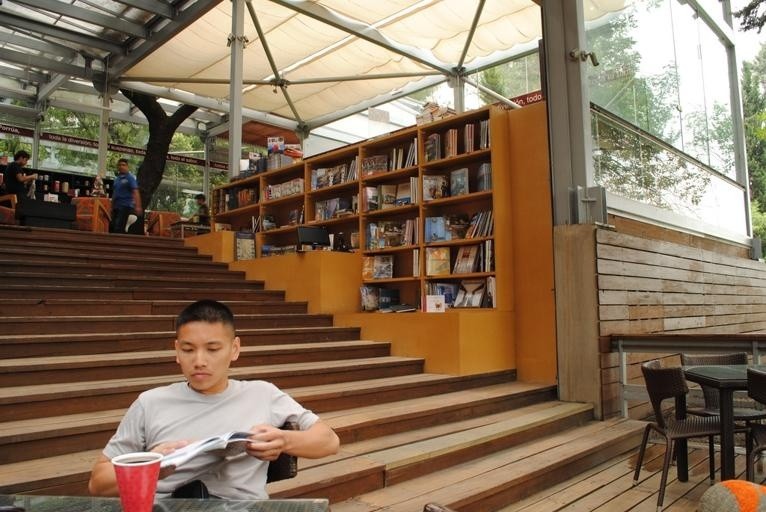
[632,361,751,512]
[76,198,111,232]
[267,421,299,483]
[0,194,17,224]
[146,212,180,237]
[422,502,459,512]
[745,369,766,485]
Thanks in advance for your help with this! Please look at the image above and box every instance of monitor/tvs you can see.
[298,226,331,246]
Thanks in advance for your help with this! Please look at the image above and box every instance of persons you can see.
[4,149,38,201]
[112,158,143,233]
[88,299,340,500]
[194,194,210,216]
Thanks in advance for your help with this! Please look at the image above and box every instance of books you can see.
[212,182,260,261]
[310,155,359,252]
[361,120,496,313]
[157,430,265,471]
[262,178,305,255]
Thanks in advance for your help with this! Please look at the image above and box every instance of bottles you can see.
[334,231,349,252]
[19,172,50,191]
[63,182,68,192]
[55,181,60,192]
[75,179,109,197]
[0,173,3,184]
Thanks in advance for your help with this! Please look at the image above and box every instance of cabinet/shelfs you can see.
[211,107,515,375]
[0,164,114,204]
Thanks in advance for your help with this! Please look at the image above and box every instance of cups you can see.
[110,452,160,512]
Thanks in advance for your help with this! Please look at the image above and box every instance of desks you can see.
[170,221,210,238]
[0,493,330,512]
[15,198,76,230]
